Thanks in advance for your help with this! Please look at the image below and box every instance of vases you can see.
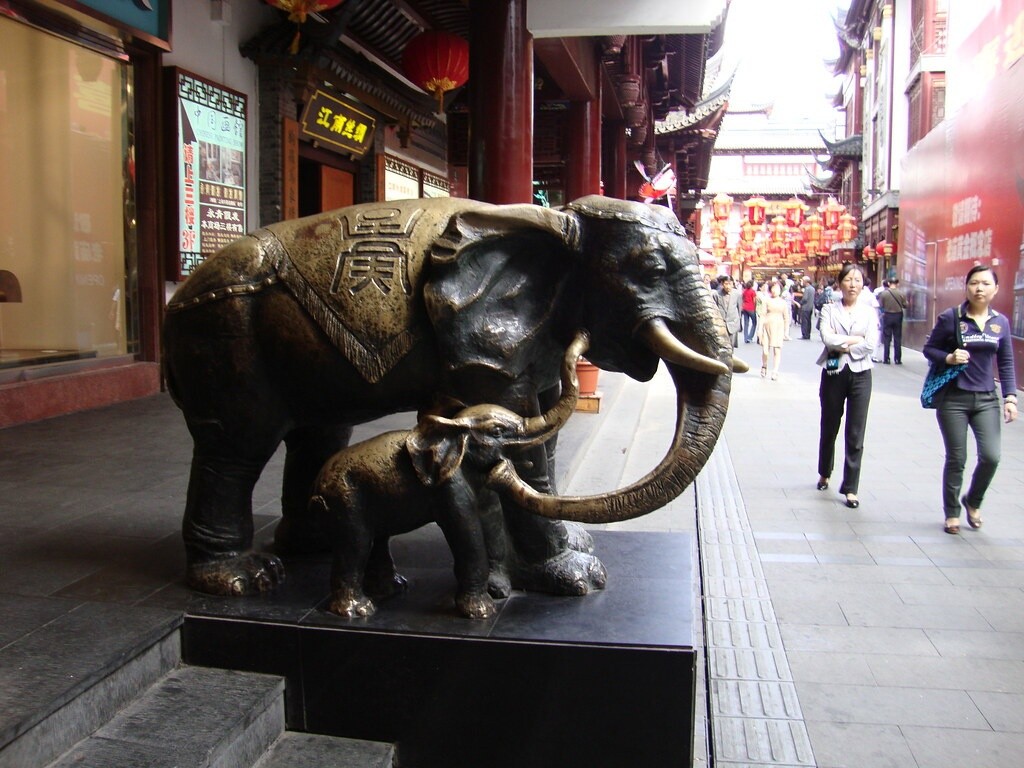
[576,359,599,396]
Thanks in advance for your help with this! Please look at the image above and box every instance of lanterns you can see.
[639,168,677,204]
[401,29,469,113]
[695,191,898,271]
[264,0,343,56]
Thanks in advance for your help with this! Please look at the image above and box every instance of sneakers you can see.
[872,358,882,362]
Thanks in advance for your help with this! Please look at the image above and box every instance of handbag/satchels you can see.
[921,362,969,408]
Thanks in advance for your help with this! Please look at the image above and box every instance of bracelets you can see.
[1004,397,1017,407]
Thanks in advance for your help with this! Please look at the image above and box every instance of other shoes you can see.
[961,495,982,529]
[883,362,889,364]
[945,519,960,534]
[771,373,778,380]
[895,362,902,364]
[761,364,767,377]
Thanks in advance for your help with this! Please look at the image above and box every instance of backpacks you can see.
[814,290,829,311]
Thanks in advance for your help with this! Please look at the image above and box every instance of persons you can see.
[919,265,1018,535]
[816,264,882,508]
[704,270,909,381]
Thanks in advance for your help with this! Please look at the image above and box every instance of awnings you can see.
[699,248,723,264]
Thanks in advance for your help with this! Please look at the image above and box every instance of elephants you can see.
[305,330,591,622]
[158,195,750,600]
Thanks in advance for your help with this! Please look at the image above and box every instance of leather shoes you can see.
[818,482,828,490]
[846,499,859,508]
